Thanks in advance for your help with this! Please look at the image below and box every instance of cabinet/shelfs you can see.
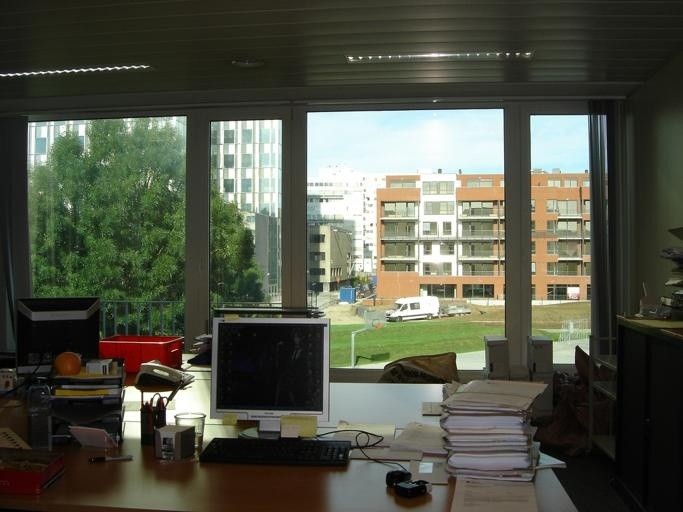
[587,335,617,467]
[612,317,682,511]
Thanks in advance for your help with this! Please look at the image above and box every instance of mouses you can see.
[386,469,412,487]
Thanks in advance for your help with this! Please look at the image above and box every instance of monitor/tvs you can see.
[15,296,101,378]
[209,317,331,439]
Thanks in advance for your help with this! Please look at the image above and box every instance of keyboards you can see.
[199,437,351,467]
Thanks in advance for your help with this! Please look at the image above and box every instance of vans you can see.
[383,296,440,321]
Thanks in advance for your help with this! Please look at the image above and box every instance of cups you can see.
[1,369,16,393]
[175,413,206,449]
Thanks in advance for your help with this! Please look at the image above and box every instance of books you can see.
[440,379,547,483]
[54,365,126,441]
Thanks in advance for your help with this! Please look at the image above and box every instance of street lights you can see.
[217,281,225,306]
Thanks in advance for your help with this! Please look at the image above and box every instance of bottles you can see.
[27,376,53,448]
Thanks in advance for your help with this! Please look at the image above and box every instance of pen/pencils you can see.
[142,380,184,444]
[89,455,133,462]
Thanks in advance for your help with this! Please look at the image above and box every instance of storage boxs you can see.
[99,337,184,372]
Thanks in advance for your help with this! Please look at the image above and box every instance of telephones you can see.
[135,359,195,388]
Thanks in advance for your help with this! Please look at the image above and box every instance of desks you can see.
[2,371,581,511]
